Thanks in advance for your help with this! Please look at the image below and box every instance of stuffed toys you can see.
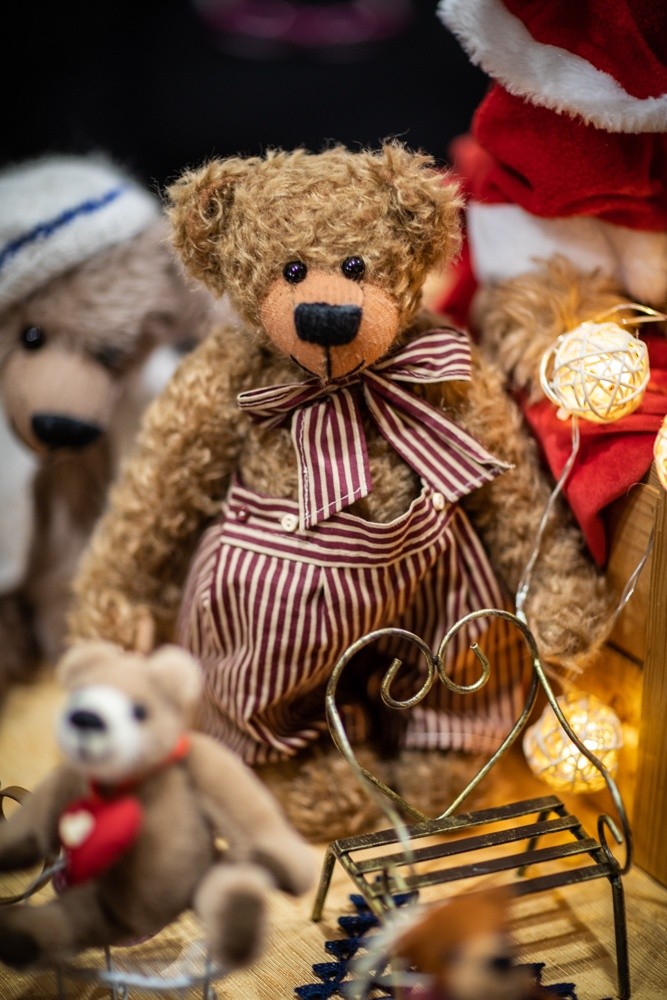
[0,149,236,685]
[62,138,614,847]
[1,639,322,971]
[404,884,557,1000]
[418,1,667,568]
